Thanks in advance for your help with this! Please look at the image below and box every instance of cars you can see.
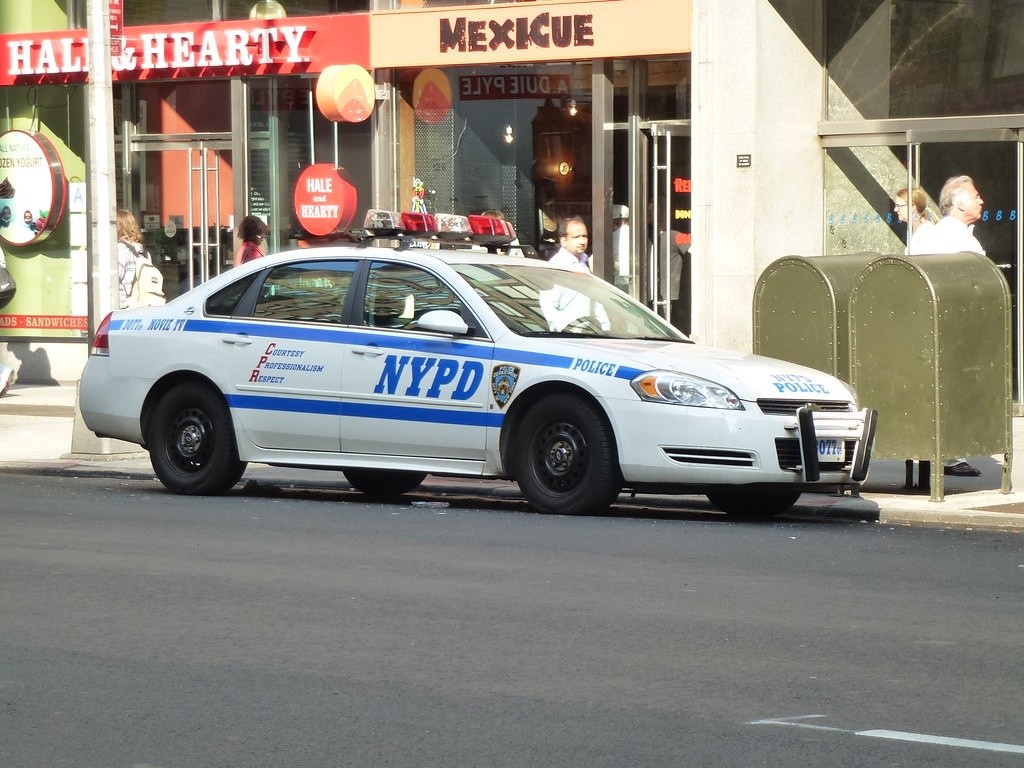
[77,210,880,523]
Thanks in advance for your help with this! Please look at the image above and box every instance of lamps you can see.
[505,125,514,143]
[569,99,578,116]
[559,161,576,176]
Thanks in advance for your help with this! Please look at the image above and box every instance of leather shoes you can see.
[945,462,981,476]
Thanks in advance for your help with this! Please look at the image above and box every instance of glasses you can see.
[894,202,908,208]
[255,234,265,238]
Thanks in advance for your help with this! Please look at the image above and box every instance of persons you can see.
[891,186,939,257]
[233,214,271,268]
[587,204,690,326]
[116,207,154,315]
[0,244,16,399]
[538,214,611,338]
[479,210,525,260]
[908,174,988,478]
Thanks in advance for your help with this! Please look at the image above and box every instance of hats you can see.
[613,204,629,220]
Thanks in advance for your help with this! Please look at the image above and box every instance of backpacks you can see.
[118,239,166,308]
